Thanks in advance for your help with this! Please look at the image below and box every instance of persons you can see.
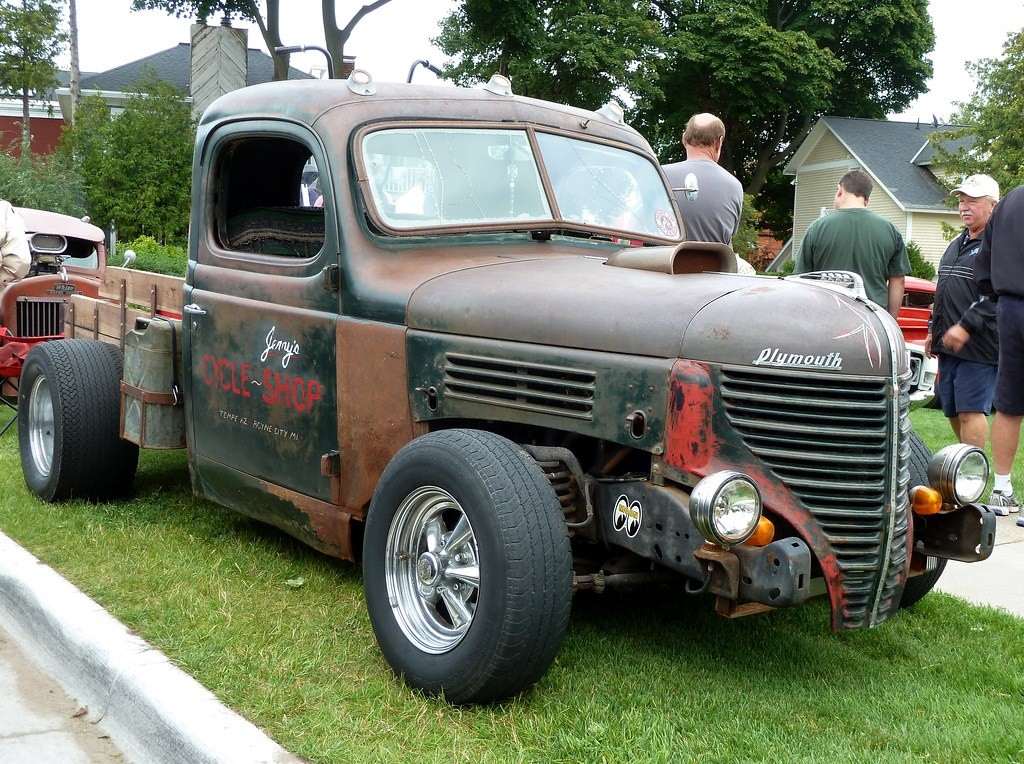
[924,170,1000,448]
[0,197,32,292]
[795,170,913,321]
[659,113,743,247]
[972,186,1024,527]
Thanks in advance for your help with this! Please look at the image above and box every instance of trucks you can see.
[0,70,996,705]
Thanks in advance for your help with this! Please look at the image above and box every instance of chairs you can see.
[0,327,65,436]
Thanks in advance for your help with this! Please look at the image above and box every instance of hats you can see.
[950,175,999,199]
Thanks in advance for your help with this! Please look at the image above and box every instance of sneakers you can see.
[1016,503,1024,527]
[987,490,1019,515]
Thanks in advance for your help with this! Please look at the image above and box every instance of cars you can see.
[895,276,938,344]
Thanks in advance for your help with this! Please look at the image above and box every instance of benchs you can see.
[227,206,428,259]
